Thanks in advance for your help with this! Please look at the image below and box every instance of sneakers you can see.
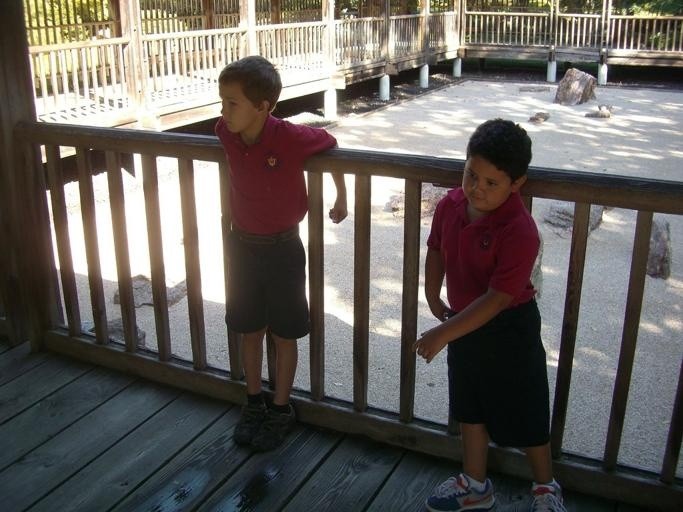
[248,404,295,451]
[234,402,267,444]
[530,479,567,512]
[425,473,494,512]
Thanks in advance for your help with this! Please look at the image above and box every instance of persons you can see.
[410,115,568,511]
[211,53,348,451]
